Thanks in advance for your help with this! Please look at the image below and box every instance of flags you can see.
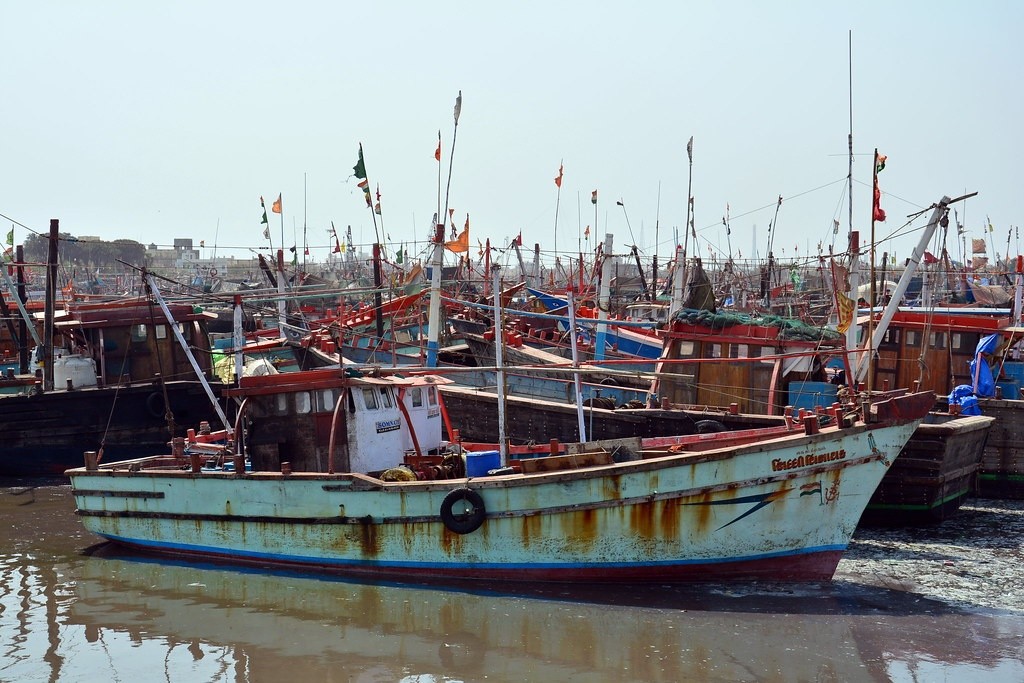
[6,229,14,246]
[272,196,281,214]
[350,148,368,178]
[442,213,469,253]
[836,291,856,335]
[289,246,296,253]
[263,225,271,241]
[972,240,986,254]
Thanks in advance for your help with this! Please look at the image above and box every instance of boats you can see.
[0,30,1024,528]
[63,263,938,584]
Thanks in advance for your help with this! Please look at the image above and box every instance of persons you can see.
[883,289,892,306]
